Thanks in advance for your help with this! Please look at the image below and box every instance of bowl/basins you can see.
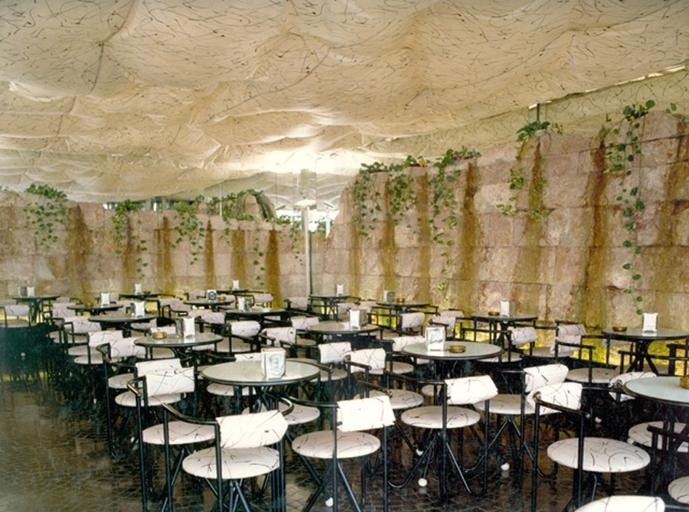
[397,297,405,303]
[613,326,627,331]
[488,311,500,316]
[679,376,689,389]
[151,332,168,339]
[448,345,466,353]
